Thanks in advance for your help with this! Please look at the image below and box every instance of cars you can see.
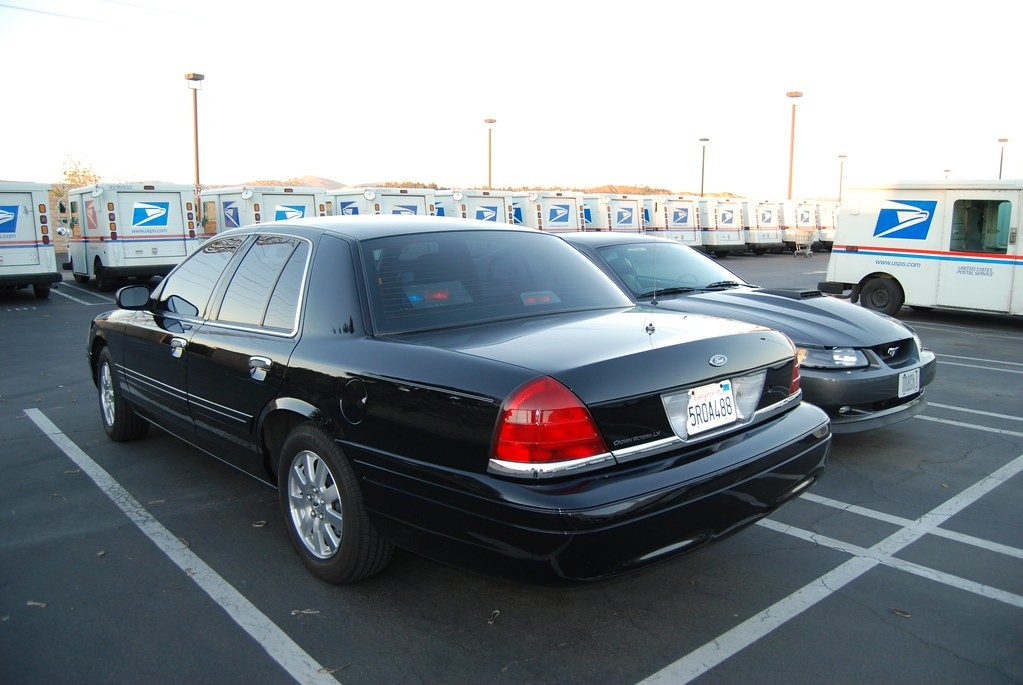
[88,215,831,584]
[552,233,938,433]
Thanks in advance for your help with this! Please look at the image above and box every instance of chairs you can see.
[412,255,451,282]
[609,257,643,291]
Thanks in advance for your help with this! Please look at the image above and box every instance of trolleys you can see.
[793,230,816,258]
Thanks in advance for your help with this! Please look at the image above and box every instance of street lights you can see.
[699,138,709,197]
[185,73,204,222]
[787,91,803,200]
[998,138,1009,179]
[838,155,847,202]
[944,169,950,181]
[485,119,496,190]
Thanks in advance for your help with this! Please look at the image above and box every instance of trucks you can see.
[325,189,438,260]
[193,187,326,245]
[818,178,1022,319]
[56,182,199,291]
[0,180,63,298]
